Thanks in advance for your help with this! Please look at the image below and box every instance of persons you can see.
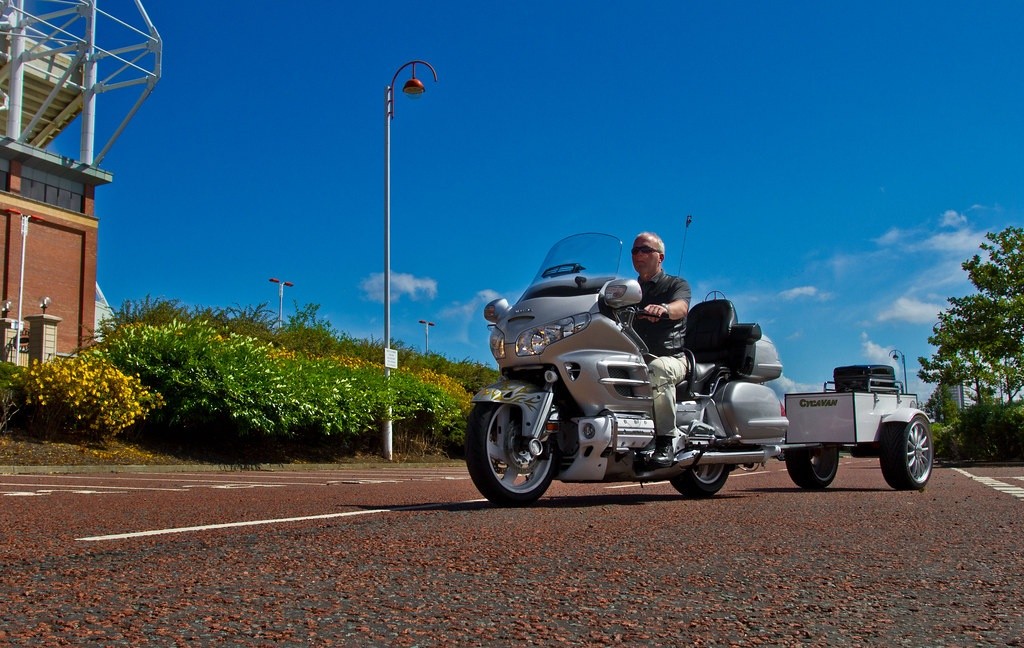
[622,232,691,464]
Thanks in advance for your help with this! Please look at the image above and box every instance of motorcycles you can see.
[464,231,790,504]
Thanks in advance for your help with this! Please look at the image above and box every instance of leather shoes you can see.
[649,443,674,467]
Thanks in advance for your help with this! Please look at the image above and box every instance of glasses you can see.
[631,246,660,254]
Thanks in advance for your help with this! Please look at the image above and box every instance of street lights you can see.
[888,350,907,395]
[382,60,437,461]
[5,207,46,365]
[419,320,435,353]
[269,278,294,328]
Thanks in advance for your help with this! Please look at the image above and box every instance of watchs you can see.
[662,303,670,312]
